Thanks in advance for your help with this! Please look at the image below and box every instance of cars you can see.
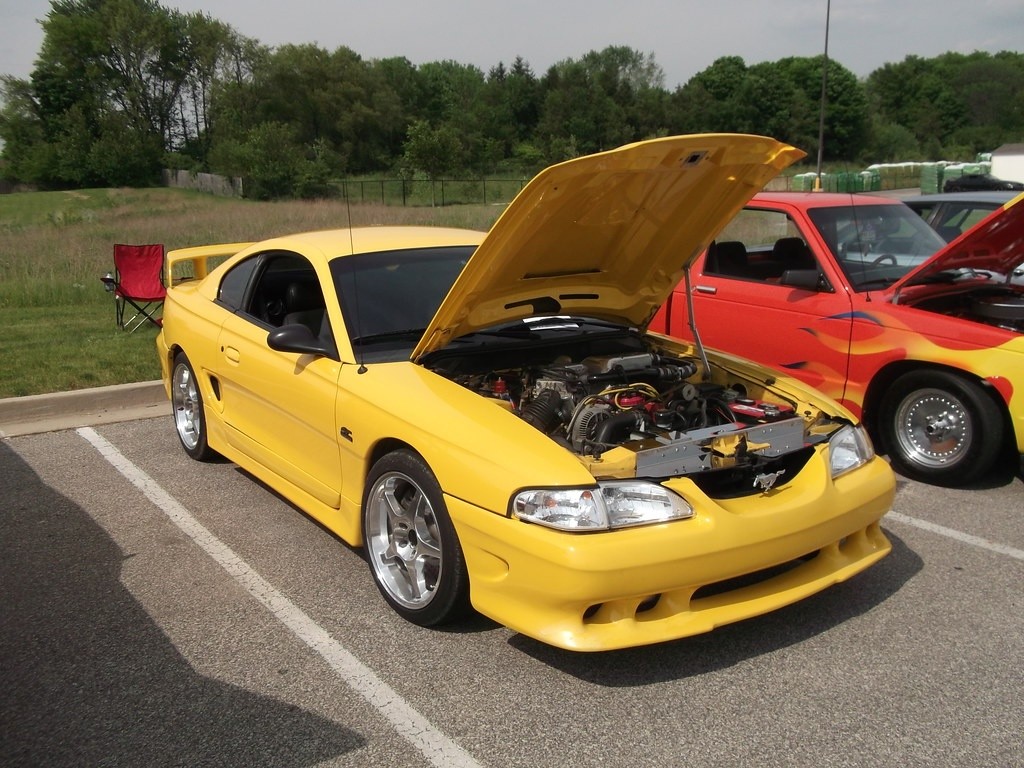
[943,174,1024,193]
[746,192,1024,288]
[156,133,896,651]
[647,191,1024,486]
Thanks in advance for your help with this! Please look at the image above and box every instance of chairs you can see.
[872,216,902,253]
[937,226,962,243]
[99,243,167,334]
[282,280,324,336]
[772,238,809,269]
[711,241,749,275]
